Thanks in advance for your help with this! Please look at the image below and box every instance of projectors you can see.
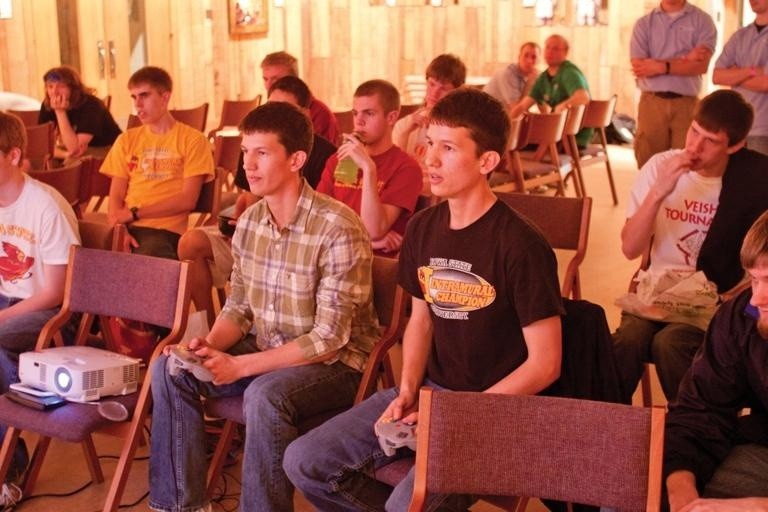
[18,345,139,404]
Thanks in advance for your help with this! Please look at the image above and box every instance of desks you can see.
[405,74,497,104]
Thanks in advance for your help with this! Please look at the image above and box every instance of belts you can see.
[654,91,683,99]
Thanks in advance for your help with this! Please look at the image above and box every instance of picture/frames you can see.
[228,0,270,34]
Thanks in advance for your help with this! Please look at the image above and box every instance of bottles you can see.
[333,129,366,186]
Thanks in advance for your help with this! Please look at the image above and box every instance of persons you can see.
[4,0,767,512]
